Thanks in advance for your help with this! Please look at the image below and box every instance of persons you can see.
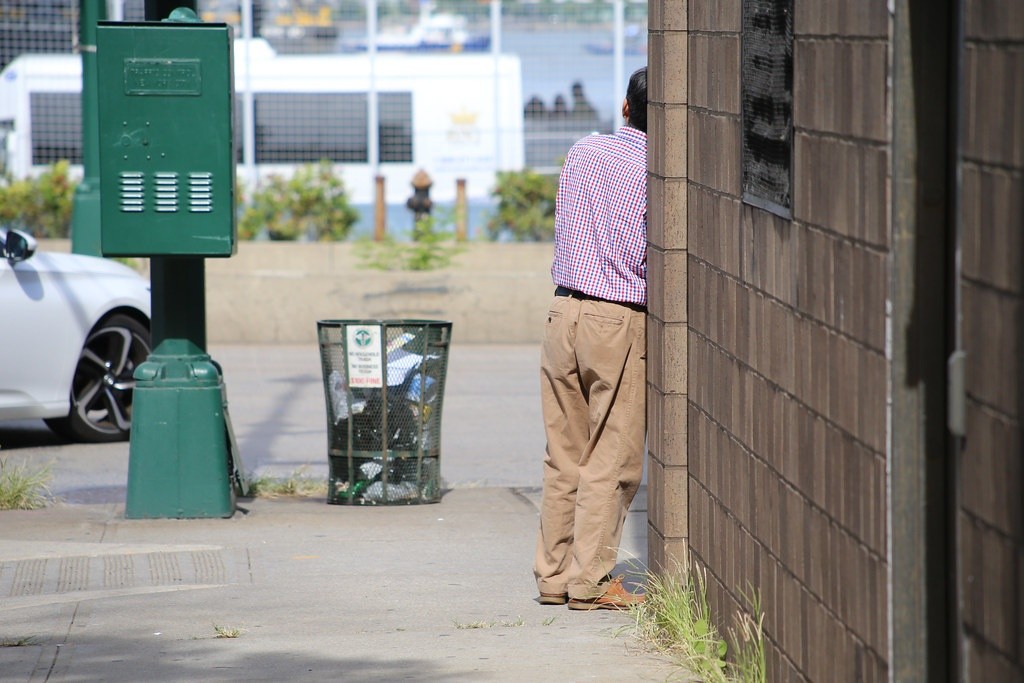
[534,67,648,610]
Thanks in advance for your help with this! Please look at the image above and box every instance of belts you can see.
[555,285,647,313]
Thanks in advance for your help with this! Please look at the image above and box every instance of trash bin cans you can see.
[317,318,453,506]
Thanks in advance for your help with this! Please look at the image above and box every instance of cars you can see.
[0,224,151,442]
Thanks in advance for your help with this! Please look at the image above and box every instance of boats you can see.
[341,0,492,53]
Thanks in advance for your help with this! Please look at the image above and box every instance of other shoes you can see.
[540,589,567,604]
[569,573,647,609]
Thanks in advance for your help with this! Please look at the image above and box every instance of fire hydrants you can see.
[405,169,433,222]
